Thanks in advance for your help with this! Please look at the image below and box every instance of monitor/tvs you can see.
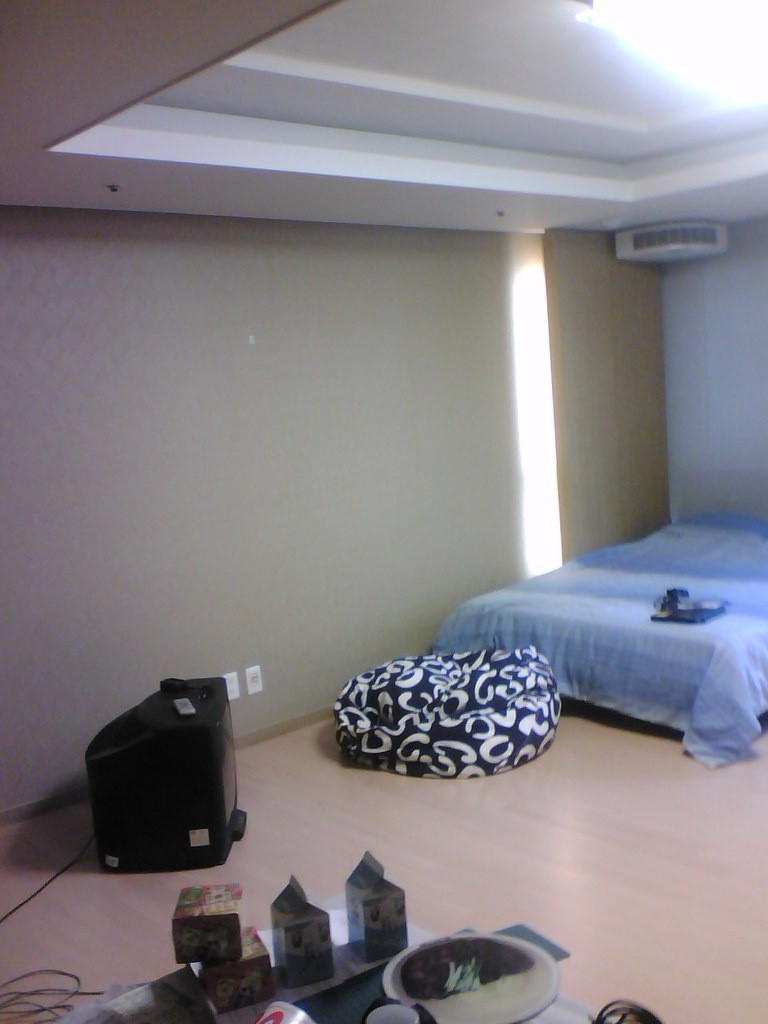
[85,676,247,875]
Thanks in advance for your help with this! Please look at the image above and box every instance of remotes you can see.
[173,697,196,716]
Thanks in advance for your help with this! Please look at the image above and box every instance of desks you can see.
[59,905,613,1024]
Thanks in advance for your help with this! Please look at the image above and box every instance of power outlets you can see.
[246,664,263,696]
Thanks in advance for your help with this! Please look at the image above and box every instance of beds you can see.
[430,509,768,769]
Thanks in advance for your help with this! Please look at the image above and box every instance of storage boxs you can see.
[171,884,244,964]
[198,927,274,1014]
